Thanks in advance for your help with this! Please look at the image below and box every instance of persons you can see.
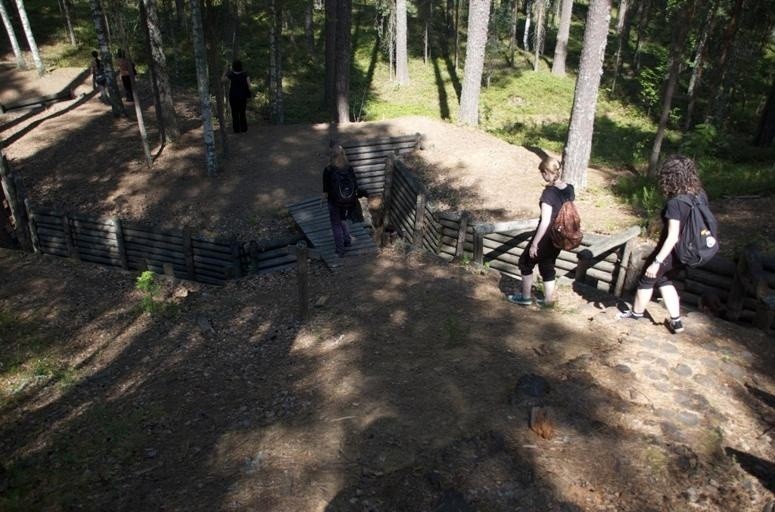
[321,144,359,258]
[220,59,251,134]
[620,154,709,334]
[508,157,575,310]
[114,48,134,101]
[90,51,108,103]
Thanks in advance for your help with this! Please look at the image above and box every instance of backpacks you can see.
[331,165,355,204]
[549,186,583,249]
[675,193,718,269]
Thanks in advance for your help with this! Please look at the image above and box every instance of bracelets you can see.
[655,256,662,264]
[531,244,539,249]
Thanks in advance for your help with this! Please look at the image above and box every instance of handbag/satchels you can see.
[94,74,106,85]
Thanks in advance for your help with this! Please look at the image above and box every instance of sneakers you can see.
[508,295,532,305]
[667,318,684,332]
[617,310,642,320]
[536,299,554,312]
